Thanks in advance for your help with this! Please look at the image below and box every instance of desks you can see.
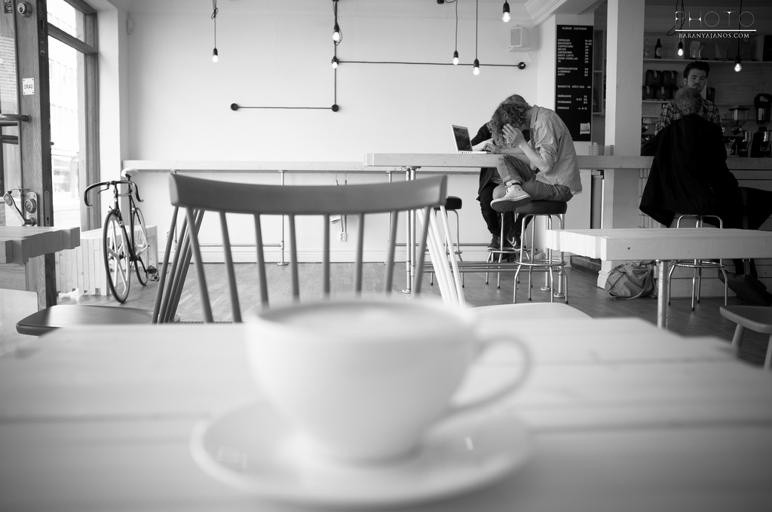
[540,225,772,329]
[0,223,81,305]
[365,154,772,229]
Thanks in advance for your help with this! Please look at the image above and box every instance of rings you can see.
[509,136,512,141]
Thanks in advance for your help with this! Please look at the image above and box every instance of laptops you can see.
[451,124,488,154]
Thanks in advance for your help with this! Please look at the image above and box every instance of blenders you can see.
[728,104,752,157]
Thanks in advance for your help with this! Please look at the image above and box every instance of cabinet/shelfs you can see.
[642,54,772,139]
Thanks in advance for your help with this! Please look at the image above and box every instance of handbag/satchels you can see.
[604,259,657,300]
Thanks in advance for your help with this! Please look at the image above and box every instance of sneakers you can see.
[490,188,531,212]
[488,232,521,251]
[719,270,771,305]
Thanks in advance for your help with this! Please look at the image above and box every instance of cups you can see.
[244,294,531,463]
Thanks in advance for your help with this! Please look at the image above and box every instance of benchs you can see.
[77,222,163,298]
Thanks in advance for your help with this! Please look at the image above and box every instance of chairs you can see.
[15,205,205,337]
[169,172,446,324]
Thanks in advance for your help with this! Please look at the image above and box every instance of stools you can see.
[483,211,521,289]
[513,196,568,305]
[719,304,772,372]
[427,195,466,289]
[666,213,729,308]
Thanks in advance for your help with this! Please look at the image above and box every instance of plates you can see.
[189,398,536,512]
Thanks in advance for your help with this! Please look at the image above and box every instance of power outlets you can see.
[339,231,347,242]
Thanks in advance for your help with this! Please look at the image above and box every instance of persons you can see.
[470,95,533,259]
[483,101,582,213]
[639,87,772,305]
[655,61,720,136]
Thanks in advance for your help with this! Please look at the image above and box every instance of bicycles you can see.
[83,174,150,303]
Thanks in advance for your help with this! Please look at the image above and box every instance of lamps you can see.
[474,1,481,77]
[331,48,340,71]
[733,1,744,72]
[501,1,513,25]
[330,1,344,45]
[452,2,461,66]
[677,2,686,58]
[209,1,218,64]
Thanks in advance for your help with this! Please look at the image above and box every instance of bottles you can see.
[655,37,663,59]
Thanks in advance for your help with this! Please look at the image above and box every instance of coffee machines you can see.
[750,91,772,158]
